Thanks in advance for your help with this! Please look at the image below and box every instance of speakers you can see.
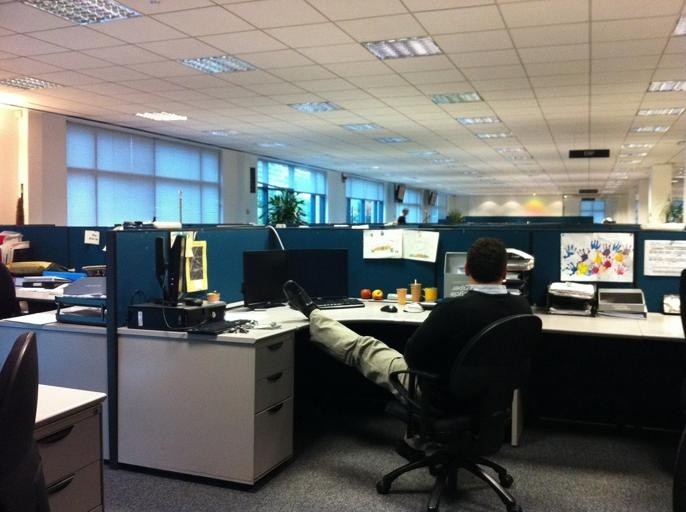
[250,168,255,193]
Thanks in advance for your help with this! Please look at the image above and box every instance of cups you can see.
[396,287,408,304]
[410,283,422,302]
[423,286,439,302]
[206,292,220,301]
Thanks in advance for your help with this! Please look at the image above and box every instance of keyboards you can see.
[311,299,365,309]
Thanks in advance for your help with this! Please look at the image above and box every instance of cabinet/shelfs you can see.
[36,383,106,512]
[115,307,301,487]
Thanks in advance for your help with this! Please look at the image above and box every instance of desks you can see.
[0,281,109,461]
[306,298,684,439]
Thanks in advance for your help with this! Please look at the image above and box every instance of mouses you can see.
[381,305,397,312]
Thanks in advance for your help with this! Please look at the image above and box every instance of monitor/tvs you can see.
[288,248,348,298]
[395,184,406,202]
[243,248,288,309]
[428,192,437,205]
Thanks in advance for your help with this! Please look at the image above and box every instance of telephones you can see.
[663,295,680,313]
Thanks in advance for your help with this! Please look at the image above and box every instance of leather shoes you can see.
[283,280,319,319]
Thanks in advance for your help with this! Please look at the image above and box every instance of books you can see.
[548,301,592,316]
[0,230,30,265]
[547,281,595,299]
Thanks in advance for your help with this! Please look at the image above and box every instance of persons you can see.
[397,208,410,224]
[283,238,535,410]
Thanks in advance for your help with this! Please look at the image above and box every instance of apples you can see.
[360,289,372,299]
[372,289,384,300]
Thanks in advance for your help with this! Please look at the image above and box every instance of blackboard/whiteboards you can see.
[560,232,637,283]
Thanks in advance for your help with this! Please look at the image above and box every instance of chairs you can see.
[0,330,51,511]
[377,313,544,511]
